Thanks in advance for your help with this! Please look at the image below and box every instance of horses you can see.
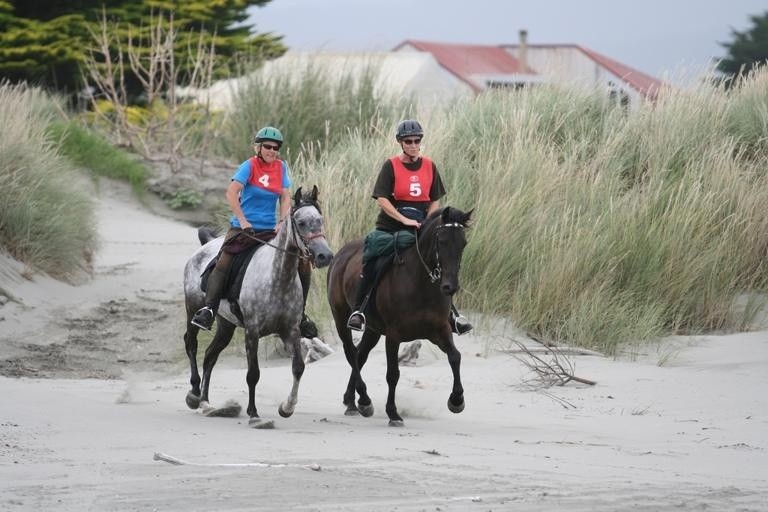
[325,206,478,429]
[182,184,335,426]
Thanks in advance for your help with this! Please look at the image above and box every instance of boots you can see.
[191,268,230,330]
[449,311,473,333]
[299,270,318,340]
[349,315,361,329]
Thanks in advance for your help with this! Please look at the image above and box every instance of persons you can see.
[188,126,321,332]
[348,119,474,336]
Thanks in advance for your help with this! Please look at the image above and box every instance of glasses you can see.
[402,139,421,144]
[262,144,279,150]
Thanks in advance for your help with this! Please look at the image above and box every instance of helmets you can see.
[395,120,424,142]
[255,126,284,147]
